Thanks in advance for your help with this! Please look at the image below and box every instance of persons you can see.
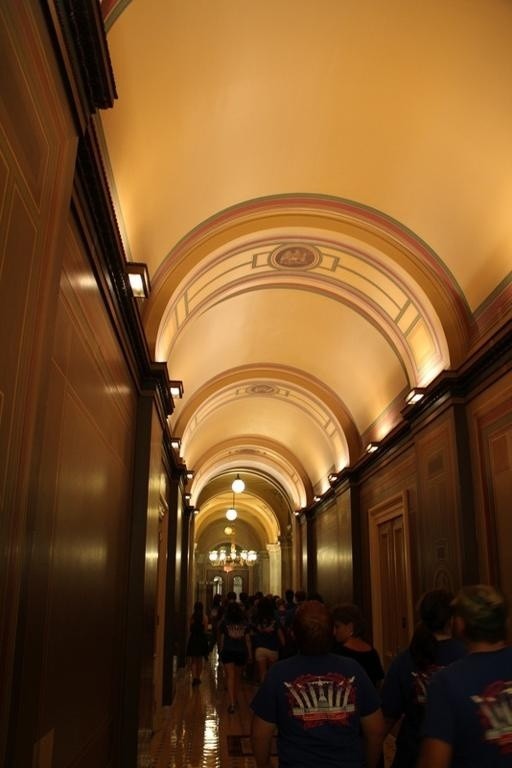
[376,589,468,768]
[414,584,512,768]
[327,602,384,691]
[248,598,388,768]
[184,587,331,712]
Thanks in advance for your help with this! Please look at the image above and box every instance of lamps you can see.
[225,473,245,535]
[294,387,426,516]
[125,262,200,514]
[208,526,258,573]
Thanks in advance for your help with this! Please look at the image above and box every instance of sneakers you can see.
[228,706,236,713]
[191,678,202,688]
